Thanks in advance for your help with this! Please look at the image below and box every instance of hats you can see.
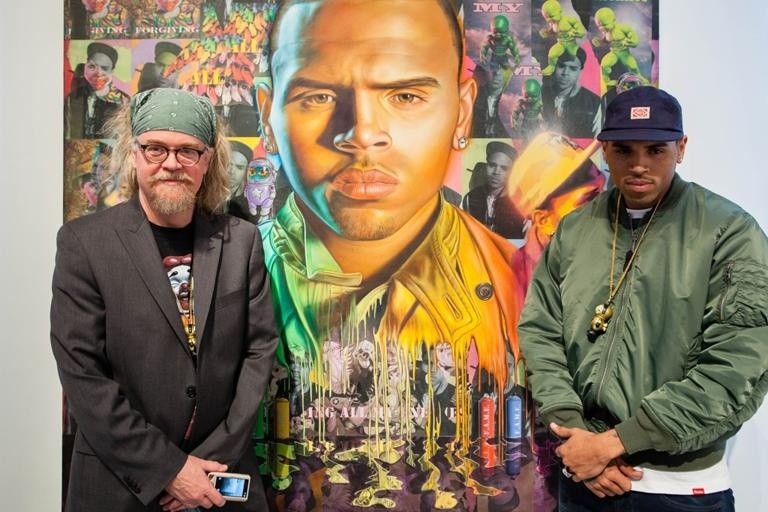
[154,41,183,58]
[86,43,118,68]
[506,131,608,219]
[486,141,517,160]
[229,139,252,161]
[597,86,683,142]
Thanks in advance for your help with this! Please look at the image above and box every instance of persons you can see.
[48,83,280,511]
[66,0,606,338]
[515,84,767,512]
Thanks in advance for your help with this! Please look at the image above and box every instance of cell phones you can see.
[208,472,251,502]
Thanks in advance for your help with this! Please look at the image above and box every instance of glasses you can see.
[134,136,209,168]
